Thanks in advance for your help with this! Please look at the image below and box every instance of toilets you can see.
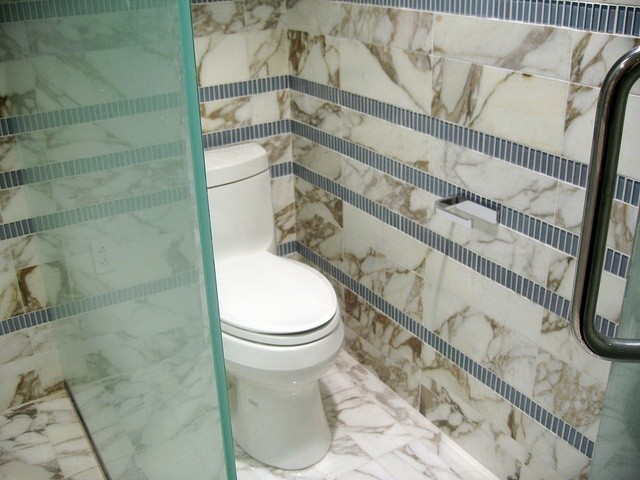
[204,141,347,472]
